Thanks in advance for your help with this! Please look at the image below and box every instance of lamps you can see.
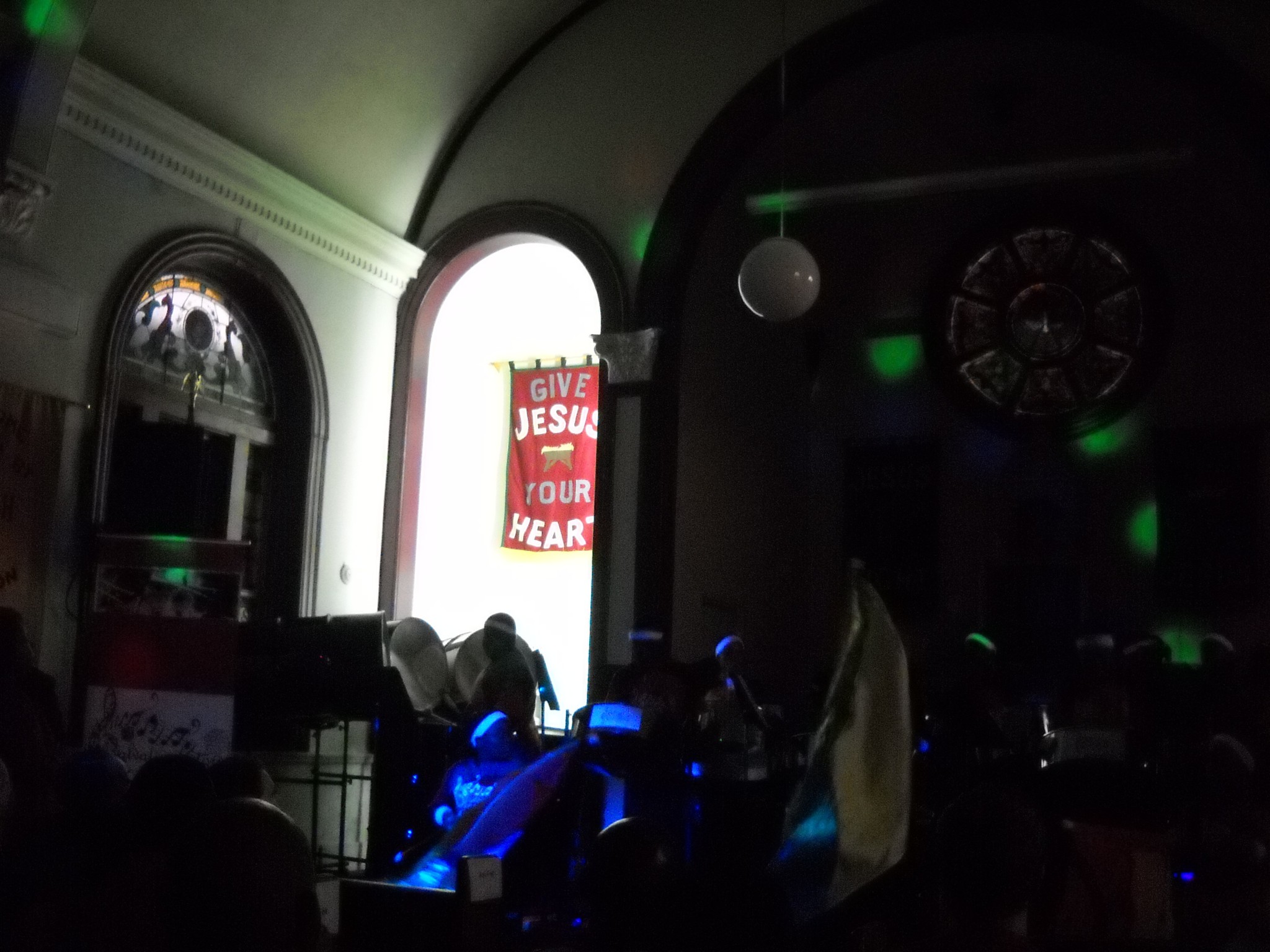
[738,2,822,322]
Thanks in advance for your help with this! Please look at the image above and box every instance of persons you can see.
[0,605,1270,952]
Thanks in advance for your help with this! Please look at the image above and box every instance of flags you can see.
[500,358,600,561]
